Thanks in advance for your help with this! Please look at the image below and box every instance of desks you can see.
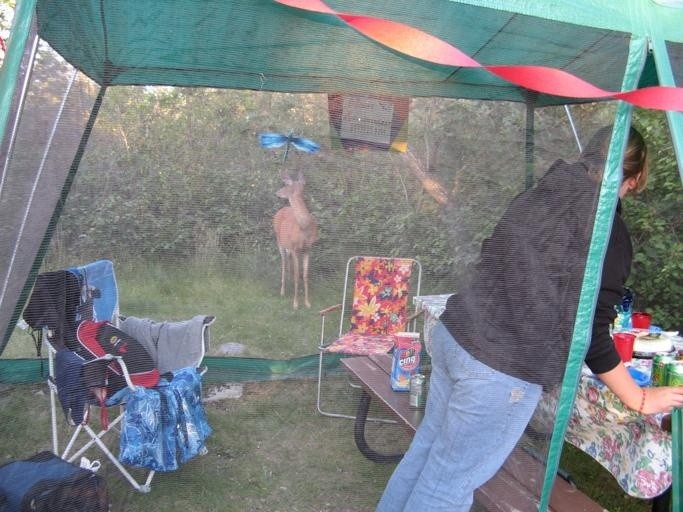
[413,294,682,501]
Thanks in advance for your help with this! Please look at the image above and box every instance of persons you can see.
[378,125,683,512]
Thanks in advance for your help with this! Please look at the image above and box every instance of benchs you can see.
[340,355,614,512]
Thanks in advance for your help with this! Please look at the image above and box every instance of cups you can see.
[609,309,654,367]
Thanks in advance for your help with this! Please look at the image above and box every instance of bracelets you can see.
[638,387,647,415]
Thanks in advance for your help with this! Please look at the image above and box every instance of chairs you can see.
[44,260,216,494]
[317,256,422,424]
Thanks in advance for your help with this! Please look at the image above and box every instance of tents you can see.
[0,0,683,512]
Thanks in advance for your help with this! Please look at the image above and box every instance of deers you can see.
[272,169,318,314]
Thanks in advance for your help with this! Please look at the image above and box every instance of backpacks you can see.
[64,319,160,392]
